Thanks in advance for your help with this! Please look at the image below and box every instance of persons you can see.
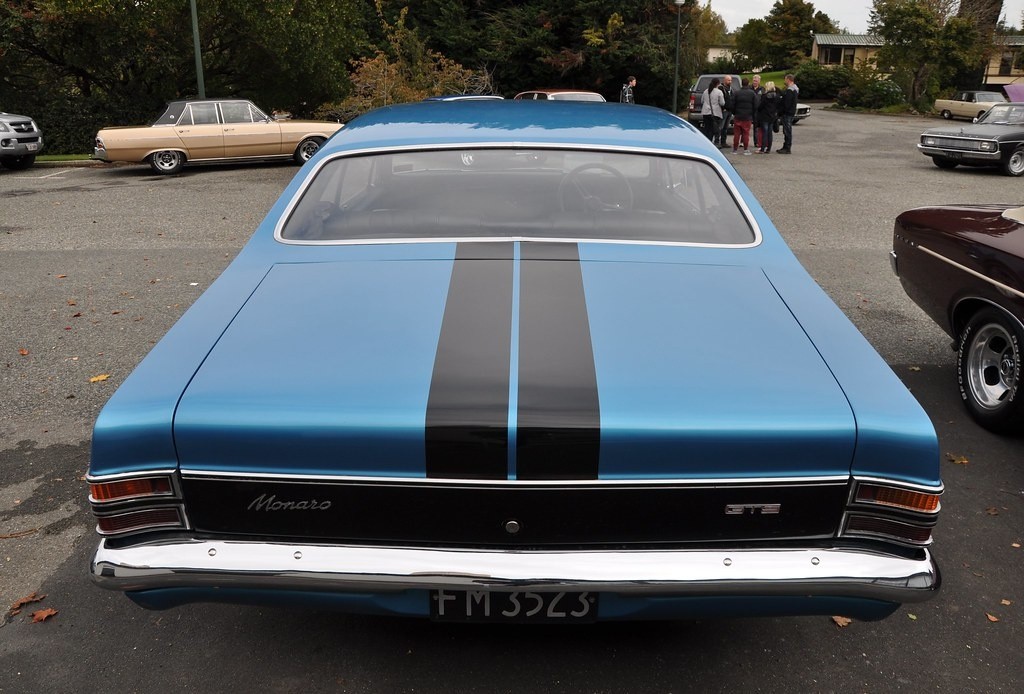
[620,76,636,105]
[719,74,734,147]
[700,78,727,149]
[775,73,799,154]
[757,80,782,152]
[739,75,765,148]
[730,77,759,155]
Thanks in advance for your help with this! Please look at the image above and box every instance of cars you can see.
[513,90,607,103]
[778,103,811,125]
[0,111,45,171]
[915,102,1024,177]
[888,202,1024,427]
[86,100,942,619]
[89,98,346,177]
[419,95,505,101]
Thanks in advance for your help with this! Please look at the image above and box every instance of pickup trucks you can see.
[933,90,1008,121]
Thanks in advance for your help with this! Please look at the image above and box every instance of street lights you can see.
[672,0,687,114]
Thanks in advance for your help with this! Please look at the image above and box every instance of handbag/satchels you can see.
[773,120,779,133]
[712,120,719,133]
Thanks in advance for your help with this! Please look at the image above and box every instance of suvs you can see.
[687,73,743,135]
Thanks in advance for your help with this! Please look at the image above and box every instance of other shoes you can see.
[755,151,764,154]
[776,149,791,154]
[744,152,752,155]
[730,152,737,154]
[722,144,730,147]
